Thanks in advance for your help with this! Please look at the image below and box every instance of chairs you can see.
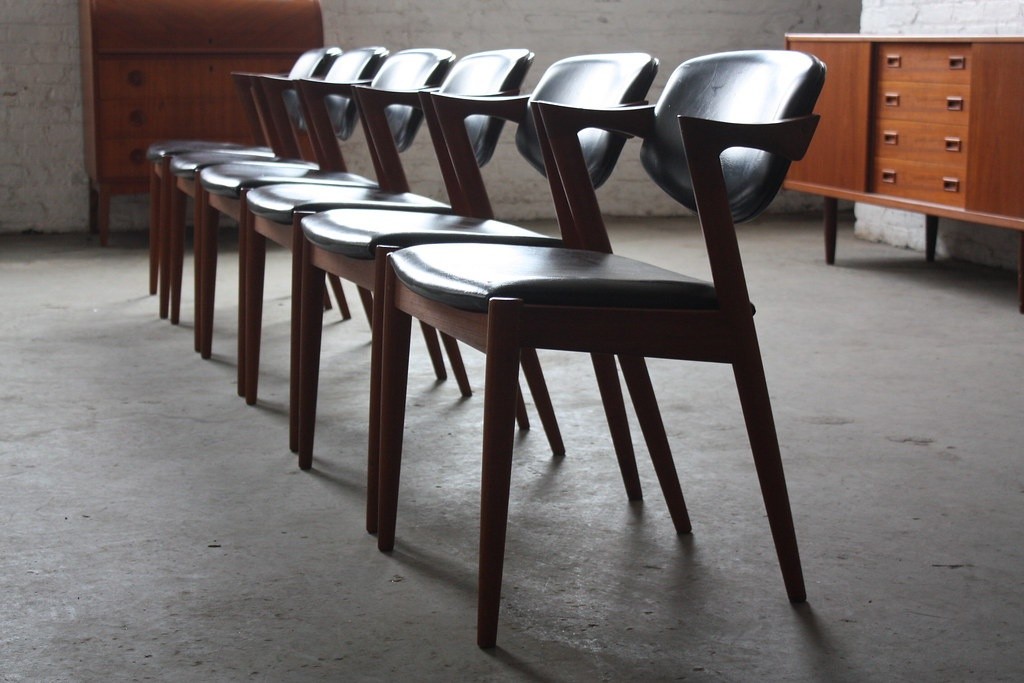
[146,46,827,650]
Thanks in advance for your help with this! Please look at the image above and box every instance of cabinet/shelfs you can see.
[77,0,331,250]
[780,34,1024,315]
